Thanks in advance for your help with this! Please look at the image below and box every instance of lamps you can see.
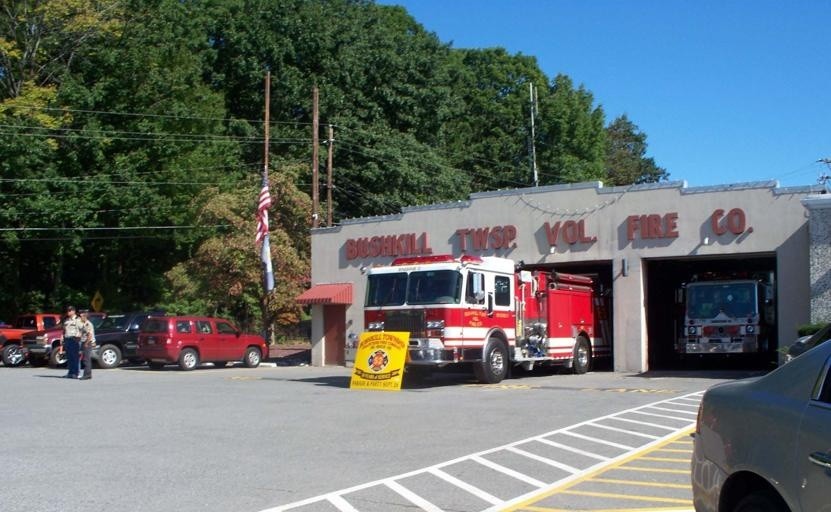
[359,266,366,273]
[703,236,709,244]
[550,246,556,254]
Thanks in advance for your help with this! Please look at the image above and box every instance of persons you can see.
[78,312,97,382]
[61,305,85,379]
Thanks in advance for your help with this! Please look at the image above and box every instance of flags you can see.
[254,170,273,246]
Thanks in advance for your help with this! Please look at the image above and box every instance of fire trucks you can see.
[674,270,776,371]
[360,254,612,384]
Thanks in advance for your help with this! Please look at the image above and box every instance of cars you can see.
[0,311,269,371]
[690,337,831,512]
